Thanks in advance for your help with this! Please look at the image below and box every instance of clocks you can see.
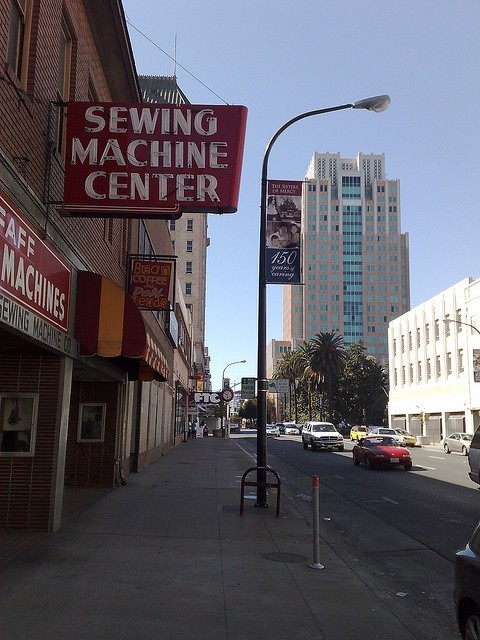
[223,390,233,400]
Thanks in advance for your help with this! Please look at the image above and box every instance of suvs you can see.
[302,422,344,452]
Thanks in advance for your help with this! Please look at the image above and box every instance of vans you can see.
[467,423,480,484]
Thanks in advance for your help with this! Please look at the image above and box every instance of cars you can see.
[395,428,417,448]
[350,426,370,442]
[455,520,480,640]
[276,423,286,431]
[369,428,407,448]
[266,426,281,438]
[443,432,473,456]
[284,423,300,435]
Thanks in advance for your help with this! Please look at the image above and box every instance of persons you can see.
[266,227,289,244]
[287,224,300,244]
[270,236,280,247]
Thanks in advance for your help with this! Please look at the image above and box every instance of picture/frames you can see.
[77,402,106,443]
[0,392,40,458]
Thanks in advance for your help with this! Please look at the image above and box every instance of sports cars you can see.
[352,436,412,470]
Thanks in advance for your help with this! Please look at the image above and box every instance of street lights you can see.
[256,94,393,508]
[443,318,480,335]
[223,360,247,434]
